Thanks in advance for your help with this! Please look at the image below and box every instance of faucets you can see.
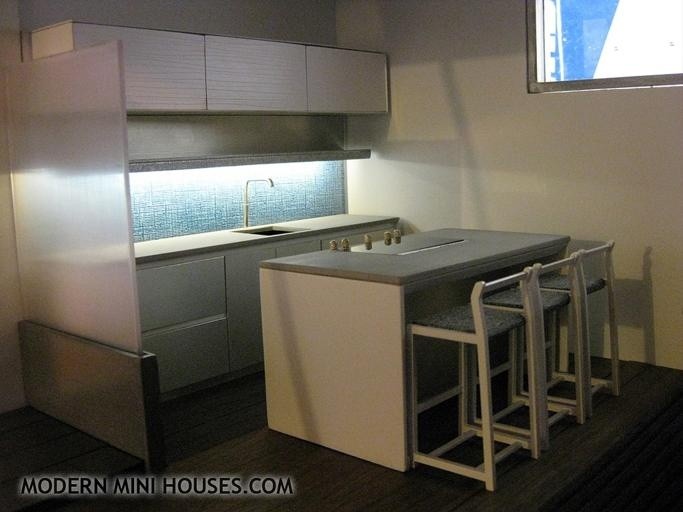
[241,178,274,226]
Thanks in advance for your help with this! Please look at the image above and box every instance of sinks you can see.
[235,225,310,237]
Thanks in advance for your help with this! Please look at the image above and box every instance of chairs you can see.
[405,239,619,494]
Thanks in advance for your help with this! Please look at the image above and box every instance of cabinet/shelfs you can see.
[225,235,322,380]
[30,22,390,116]
[134,252,228,406]
